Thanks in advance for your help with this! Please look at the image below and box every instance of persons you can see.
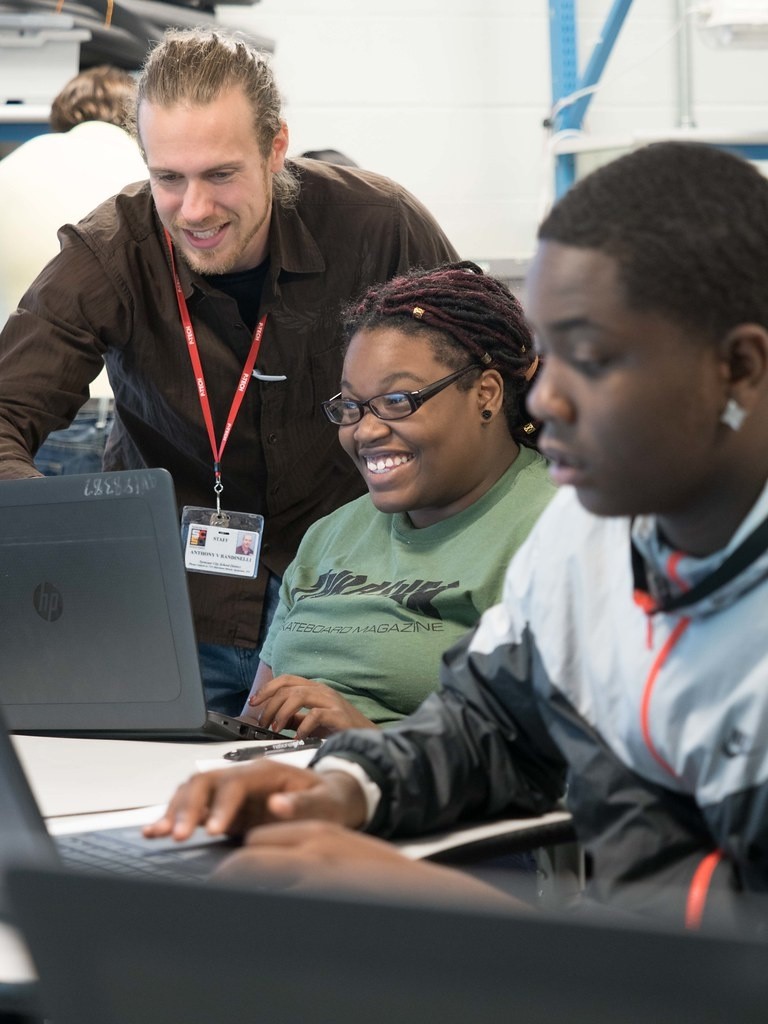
[0,64,149,332]
[0,32,473,719]
[144,140,768,937]
[235,261,561,741]
[237,535,253,555]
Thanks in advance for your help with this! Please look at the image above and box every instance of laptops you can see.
[0,726,768,1024]
[0,465,296,745]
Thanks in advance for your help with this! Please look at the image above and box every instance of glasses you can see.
[320,363,483,425]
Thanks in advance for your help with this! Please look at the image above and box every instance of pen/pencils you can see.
[223,734,326,762]
